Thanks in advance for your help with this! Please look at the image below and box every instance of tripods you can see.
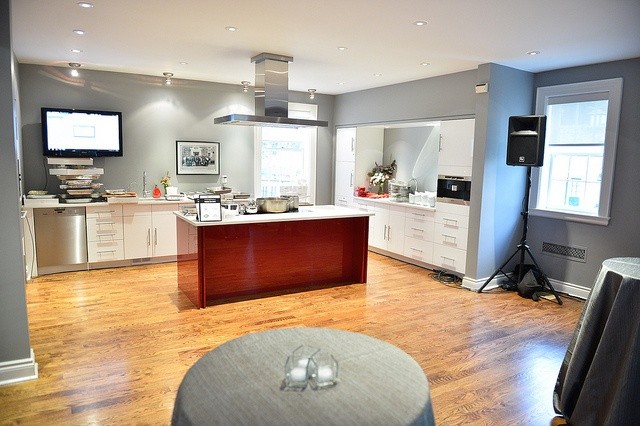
[476,168,564,304]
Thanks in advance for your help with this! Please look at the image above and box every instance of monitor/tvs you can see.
[41,107,123,157]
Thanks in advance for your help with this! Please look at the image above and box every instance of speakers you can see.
[506,115,545,166]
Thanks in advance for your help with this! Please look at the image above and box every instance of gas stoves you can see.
[223,203,299,215]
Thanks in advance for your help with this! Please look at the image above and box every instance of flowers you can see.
[160,171,176,183]
[368,160,397,186]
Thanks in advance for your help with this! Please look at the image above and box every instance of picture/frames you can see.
[176,140,221,175]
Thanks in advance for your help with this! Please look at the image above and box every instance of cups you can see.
[285,355,309,390]
[293,344,322,375]
[310,355,339,387]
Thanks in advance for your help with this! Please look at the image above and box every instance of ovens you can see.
[436,175,472,206]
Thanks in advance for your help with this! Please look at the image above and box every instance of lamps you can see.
[163,72,174,84]
[308,89,316,99]
[69,63,81,76]
[241,81,251,92]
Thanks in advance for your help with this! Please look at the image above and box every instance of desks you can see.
[171,328,435,425]
[553,257,640,425]
[176,204,366,307]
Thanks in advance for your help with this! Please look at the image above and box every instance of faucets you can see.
[143,171,147,193]
[407,178,419,192]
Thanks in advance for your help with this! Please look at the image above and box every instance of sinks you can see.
[137,192,166,200]
[394,194,409,202]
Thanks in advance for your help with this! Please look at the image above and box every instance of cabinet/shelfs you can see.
[354,199,375,249]
[85,204,125,263]
[403,207,435,265]
[375,203,405,256]
[336,128,356,162]
[337,197,353,208]
[433,212,468,275]
[439,120,475,166]
[123,203,179,263]
[336,162,356,196]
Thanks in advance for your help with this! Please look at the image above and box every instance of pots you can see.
[280,196,299,209]
[256,197,289,212]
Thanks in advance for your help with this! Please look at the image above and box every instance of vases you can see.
[379,182,386,194]
[164,184,168,194]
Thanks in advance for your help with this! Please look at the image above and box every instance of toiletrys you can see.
[153,185,161,198]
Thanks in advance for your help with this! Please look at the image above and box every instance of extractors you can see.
[214,52,328,129]
